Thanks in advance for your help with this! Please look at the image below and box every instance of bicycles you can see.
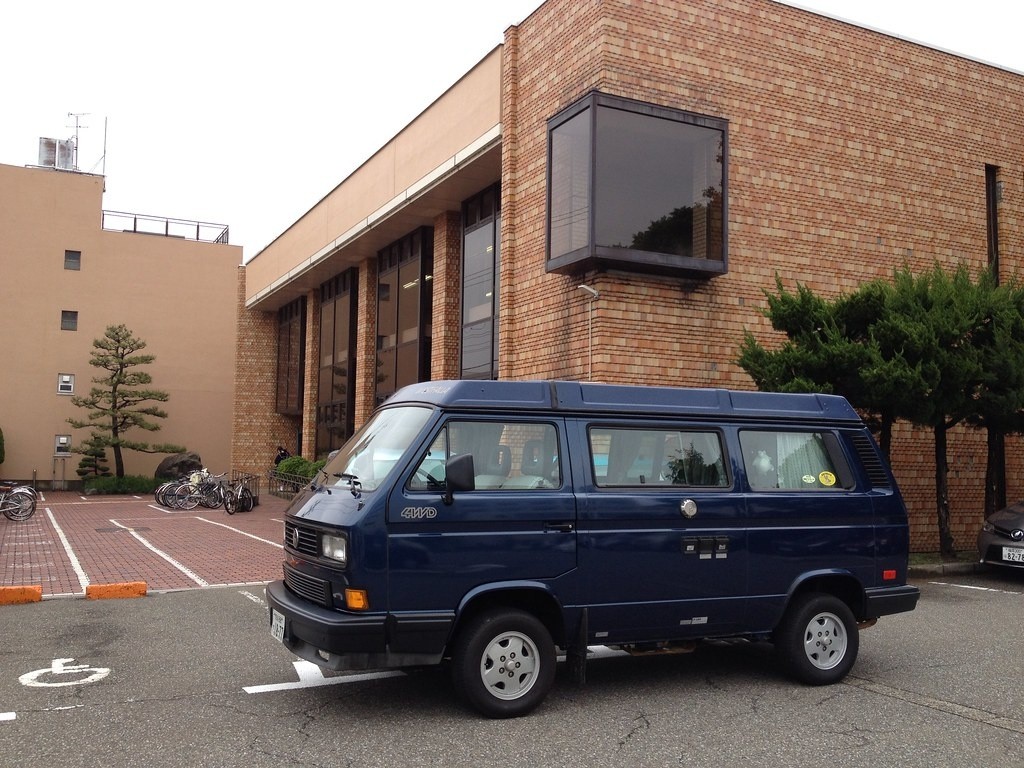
[0,481,37,522]
[154,467,254,515]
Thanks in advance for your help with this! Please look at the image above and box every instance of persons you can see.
[274,446,292,468]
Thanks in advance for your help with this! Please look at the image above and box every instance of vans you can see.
[262,380,925,718]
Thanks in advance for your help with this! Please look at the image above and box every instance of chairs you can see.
[475,445,511,490]
[502,438,556,489]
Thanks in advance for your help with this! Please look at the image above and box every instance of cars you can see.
[977,502,1024,579]
